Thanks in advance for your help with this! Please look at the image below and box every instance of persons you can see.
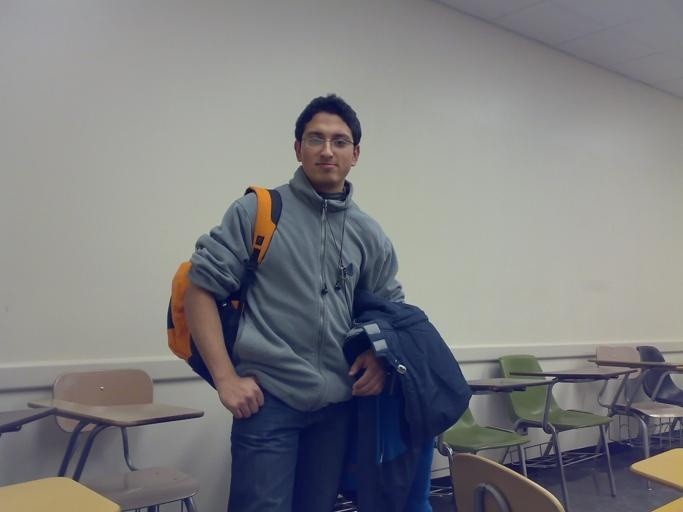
[183,92,404,512]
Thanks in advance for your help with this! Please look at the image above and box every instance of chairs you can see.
[636,346,683,404]
[588,346,683,495]
[52,367,201,512]
[449,452,567,512]
[497,354,616,512]
[432,405,530,476]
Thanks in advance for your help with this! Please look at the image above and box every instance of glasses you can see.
[301,137,356,149]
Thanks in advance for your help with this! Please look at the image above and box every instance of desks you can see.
[0,474,122,512]
[509,366,638,385]
[589,358,682,374]
[24,398,205,485]
[467,378,556,395]
[629,447,682,494]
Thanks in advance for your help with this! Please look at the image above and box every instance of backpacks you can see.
[166,183,282,389]
